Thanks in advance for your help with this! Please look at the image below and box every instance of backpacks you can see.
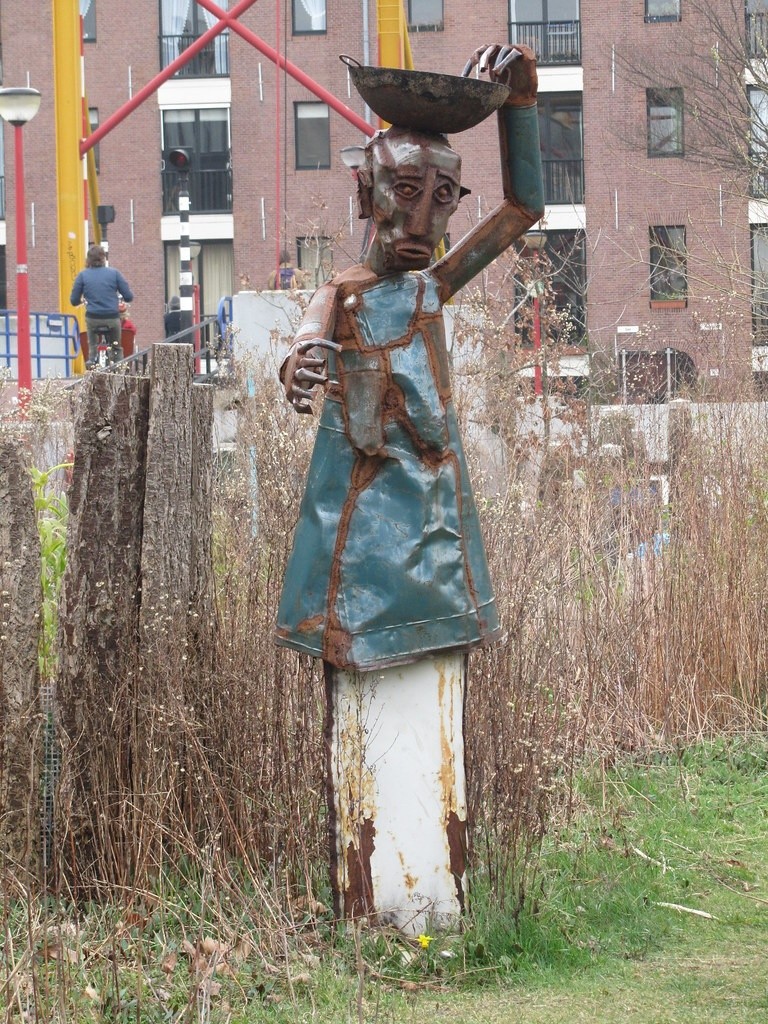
[274,268,294,290]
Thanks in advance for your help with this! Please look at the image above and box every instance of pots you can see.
[339,53,511,133]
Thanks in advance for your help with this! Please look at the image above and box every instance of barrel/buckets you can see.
[79,329,134,370]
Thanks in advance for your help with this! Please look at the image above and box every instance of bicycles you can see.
[82,294,127,374]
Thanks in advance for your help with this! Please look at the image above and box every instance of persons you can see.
[71,246,132,373]
[268,250,305,290]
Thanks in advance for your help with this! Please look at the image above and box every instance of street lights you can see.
[524,233,548,395]
[178,242,202,328]
[0,86,42,423]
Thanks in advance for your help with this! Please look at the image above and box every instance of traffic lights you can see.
[164,146,195,172]
